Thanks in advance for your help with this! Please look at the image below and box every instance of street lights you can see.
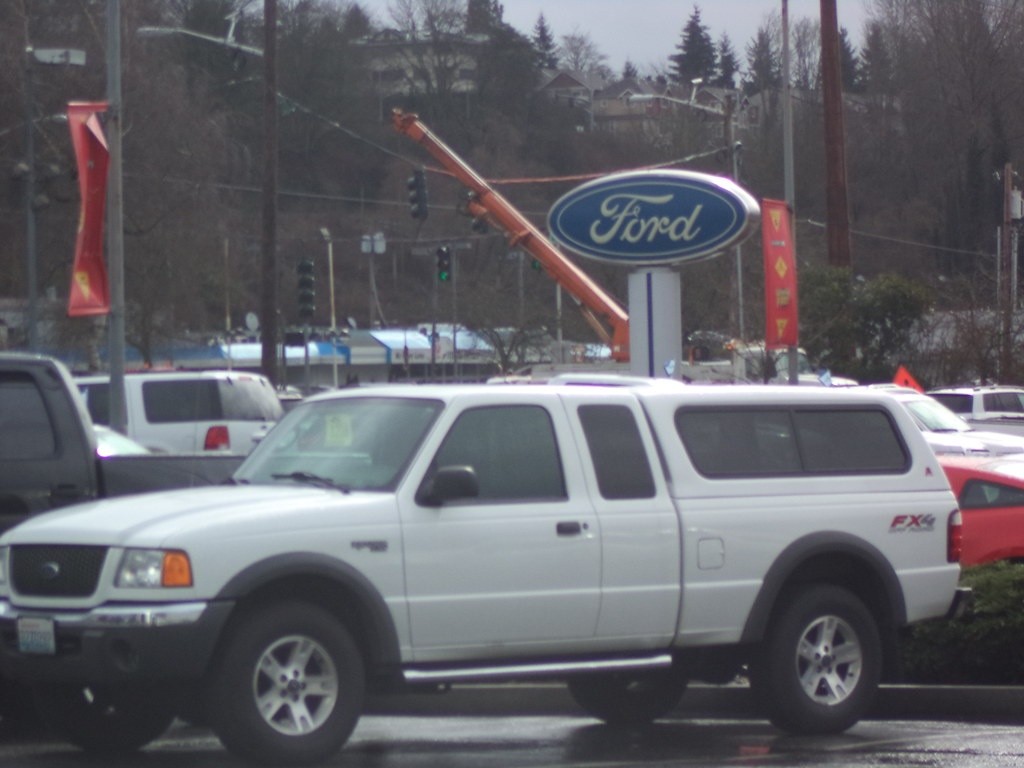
[313,222,341,395]
[627,91,747,343]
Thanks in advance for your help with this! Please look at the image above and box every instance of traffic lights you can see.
[436,245,452,283]
[296,259,312,318]
[409,166,429,222]
[467,190,488,235]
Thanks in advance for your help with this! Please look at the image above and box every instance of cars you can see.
[63,372,292,462]
[484,372,678,388]
[2,351,102,537]
[754,372,1024,572]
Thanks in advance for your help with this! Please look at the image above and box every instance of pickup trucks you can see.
[0,375,966,764]
